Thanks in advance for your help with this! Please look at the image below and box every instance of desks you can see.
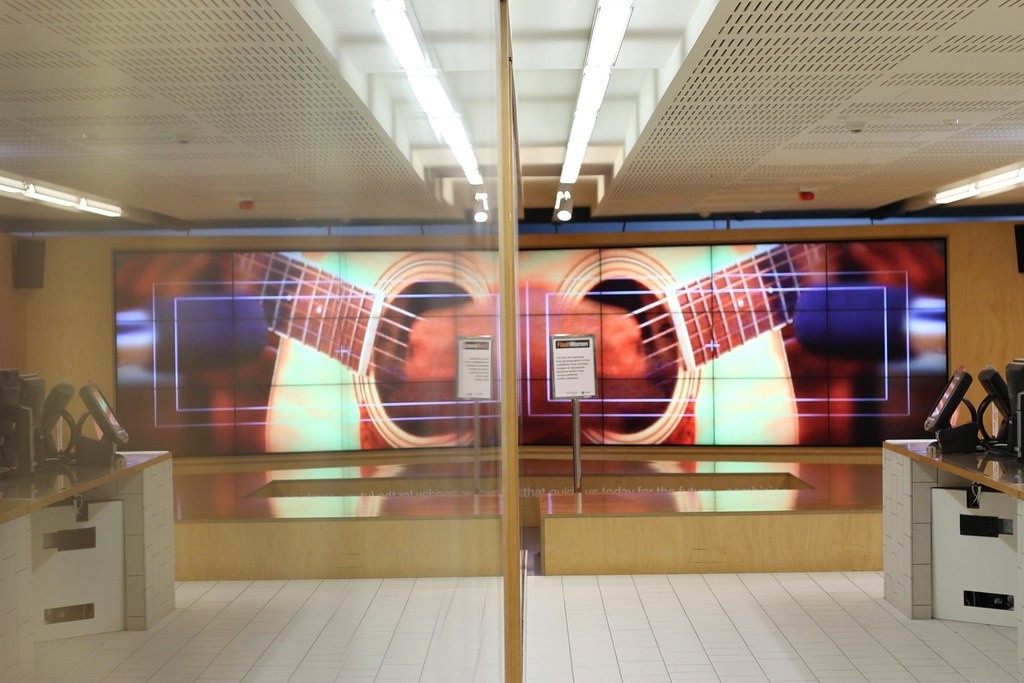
[0,451,174,683]
[883,440,1024,620]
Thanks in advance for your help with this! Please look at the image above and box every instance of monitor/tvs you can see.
[923,359,1024,463]
[0,367,129,478]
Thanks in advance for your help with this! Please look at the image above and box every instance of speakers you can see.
[14,239,46,289]
